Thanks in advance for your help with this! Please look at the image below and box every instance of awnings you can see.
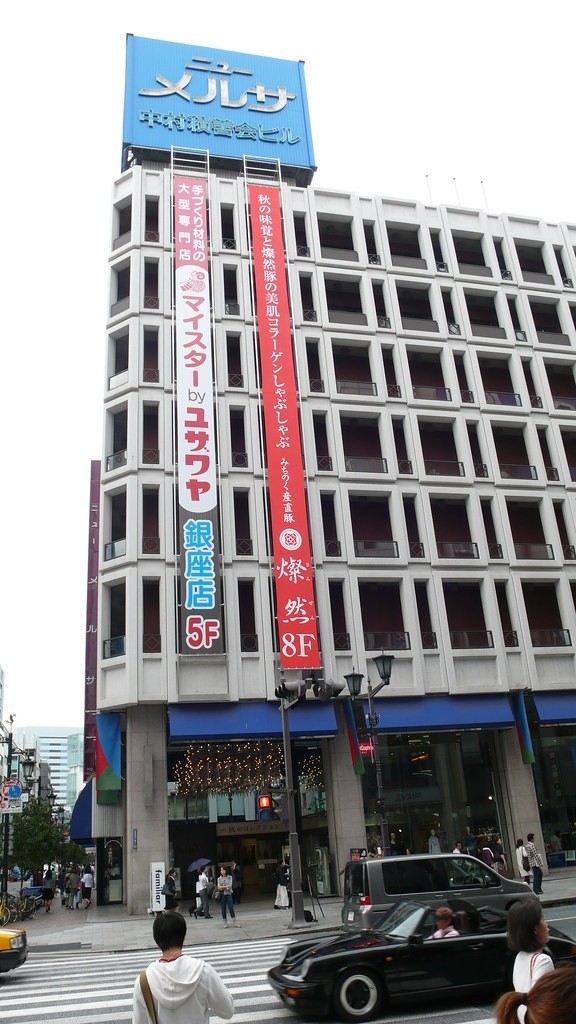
[70,778,96,848]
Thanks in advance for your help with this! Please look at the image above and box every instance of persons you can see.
[428,829,441,853]
[164,869,180,912]
[42,864,95,912]
[476,835,508,873]
[453,841,461,854]
[390,832,402,855]
[274,859,293,909]
[188,864,242,927]
[497,898,576,1024]
[516,833,543,894]
[132,911,234,1024]
[425,906,461,939]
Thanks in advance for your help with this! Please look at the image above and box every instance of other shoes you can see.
[204,915,213,919]
[535,891,544,894]
[224,924,228,928]
[45,904,51,912]
[234,925,240,928]
[60,898,93,910]
[193,911,197,919]
[188,908,193,917]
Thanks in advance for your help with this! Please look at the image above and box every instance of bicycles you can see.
[0,891,38,926]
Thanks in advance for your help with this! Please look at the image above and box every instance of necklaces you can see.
[160,955,183,962]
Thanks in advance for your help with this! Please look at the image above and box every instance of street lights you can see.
[38,787,57,805]
[343,647,396,857]
[1,750,37,890]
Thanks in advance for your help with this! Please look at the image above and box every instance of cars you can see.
[12,869,26,881]
[0,926,29,974]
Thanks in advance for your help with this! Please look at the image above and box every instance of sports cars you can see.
[266,898,576,1022]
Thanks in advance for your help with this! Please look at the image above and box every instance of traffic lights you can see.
[258,795,274,822]
[318,679,346,699]
[274,680,306,700]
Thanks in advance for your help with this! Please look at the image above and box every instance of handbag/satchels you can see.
[215,892,221,899]
[161,885,176,896]
[303,910,314,922]
[522,857,531,871]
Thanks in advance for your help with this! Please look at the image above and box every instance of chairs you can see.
[453,912,468,936]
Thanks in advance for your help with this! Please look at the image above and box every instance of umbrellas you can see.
[187,858,212,872]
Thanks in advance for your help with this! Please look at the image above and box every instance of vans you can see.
[341,854,540,931]
